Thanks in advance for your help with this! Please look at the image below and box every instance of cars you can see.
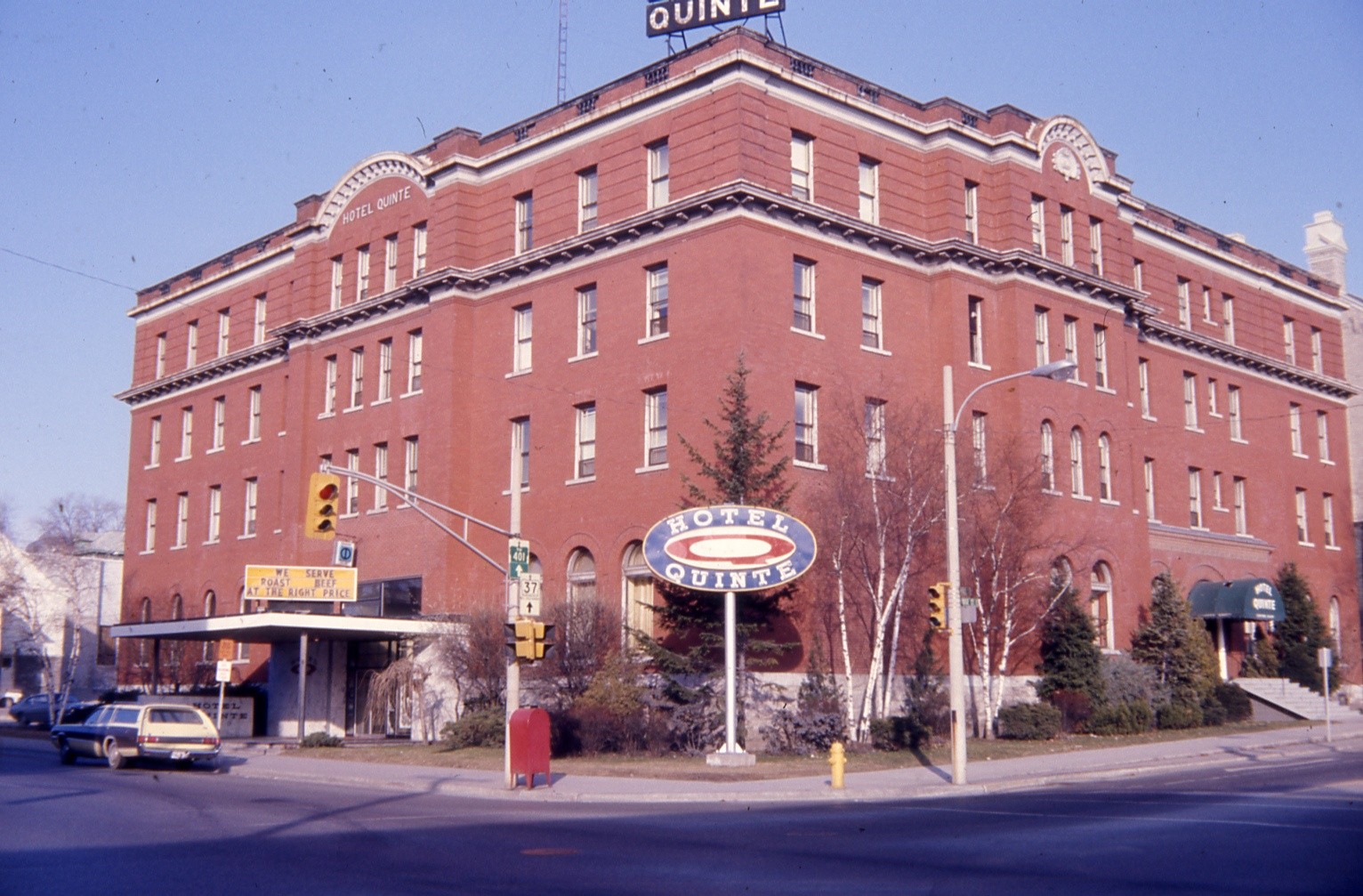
[8,692,84,726]
[52,703,222,773]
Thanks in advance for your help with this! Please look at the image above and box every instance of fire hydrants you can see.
[827,739,848,789]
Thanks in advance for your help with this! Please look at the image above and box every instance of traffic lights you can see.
[305,470,342,543]
[928,585,946,628]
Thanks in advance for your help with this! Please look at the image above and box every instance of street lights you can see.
[938,357,1082,789]
